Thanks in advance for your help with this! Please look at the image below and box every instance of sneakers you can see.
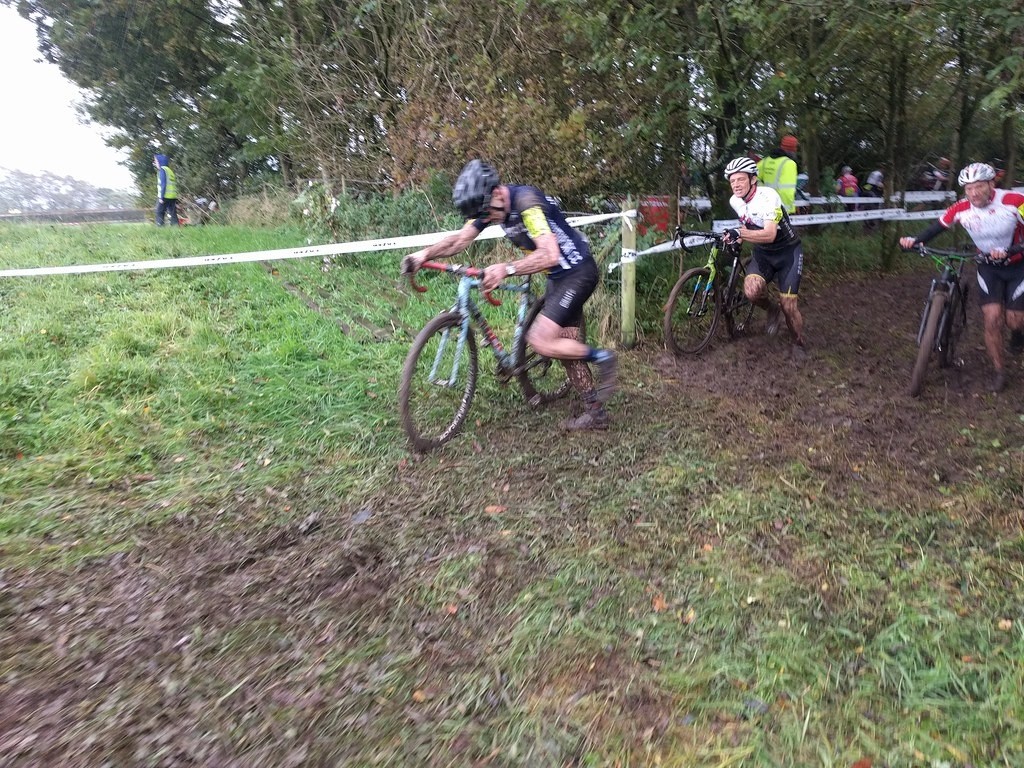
[558,407,609,431]
[596,348,618,400]
[766,300,781,337]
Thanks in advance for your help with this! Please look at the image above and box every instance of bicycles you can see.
[395,257,571,453]
[662,226,790,359]
[897,238,1024,398]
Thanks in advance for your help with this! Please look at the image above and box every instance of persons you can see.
[154,155,179,226]
[861,170,884,236]
[722,157,807,370]
[756,136,798,215]
[910,157,951,212]
[900,163,1024,393]
[400,160,618,430]
[837,167,858,228]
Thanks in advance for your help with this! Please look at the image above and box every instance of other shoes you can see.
[1010,330,1024,355]
[989,372,1007,392]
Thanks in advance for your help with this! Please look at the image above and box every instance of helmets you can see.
[940,157,952,166]
[958,161,996,187]
[724,157,759,180]
[452,159,500,209]
[842,166,853,173]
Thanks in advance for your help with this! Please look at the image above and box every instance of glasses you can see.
[478,206,492,219]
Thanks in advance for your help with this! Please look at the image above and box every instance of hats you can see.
[780,136,798,154]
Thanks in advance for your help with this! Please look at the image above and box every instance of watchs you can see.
[506,262,516,277]
